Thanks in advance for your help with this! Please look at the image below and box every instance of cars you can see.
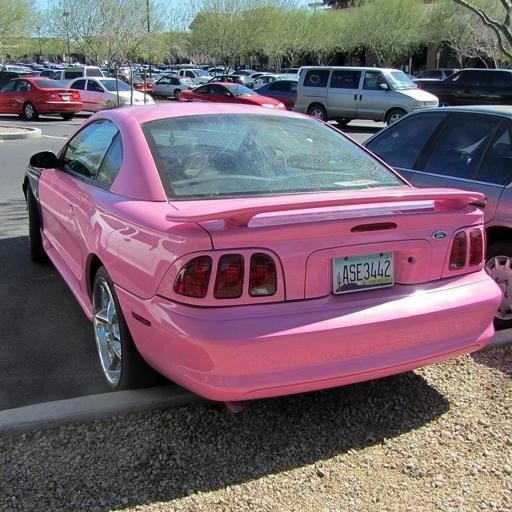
[252,81,298,109]
[0,61,299,100]
[65,76,155,113]
[410,68,512,107]
[360,105,512,329]
[0,76,84,121]
[177,82,287,111]
[22,102,503,403]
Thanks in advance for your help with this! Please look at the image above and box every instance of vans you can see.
[294,65,439,127]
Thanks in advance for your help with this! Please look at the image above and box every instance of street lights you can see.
[62,10,71,64]
[36,27,42,65]
